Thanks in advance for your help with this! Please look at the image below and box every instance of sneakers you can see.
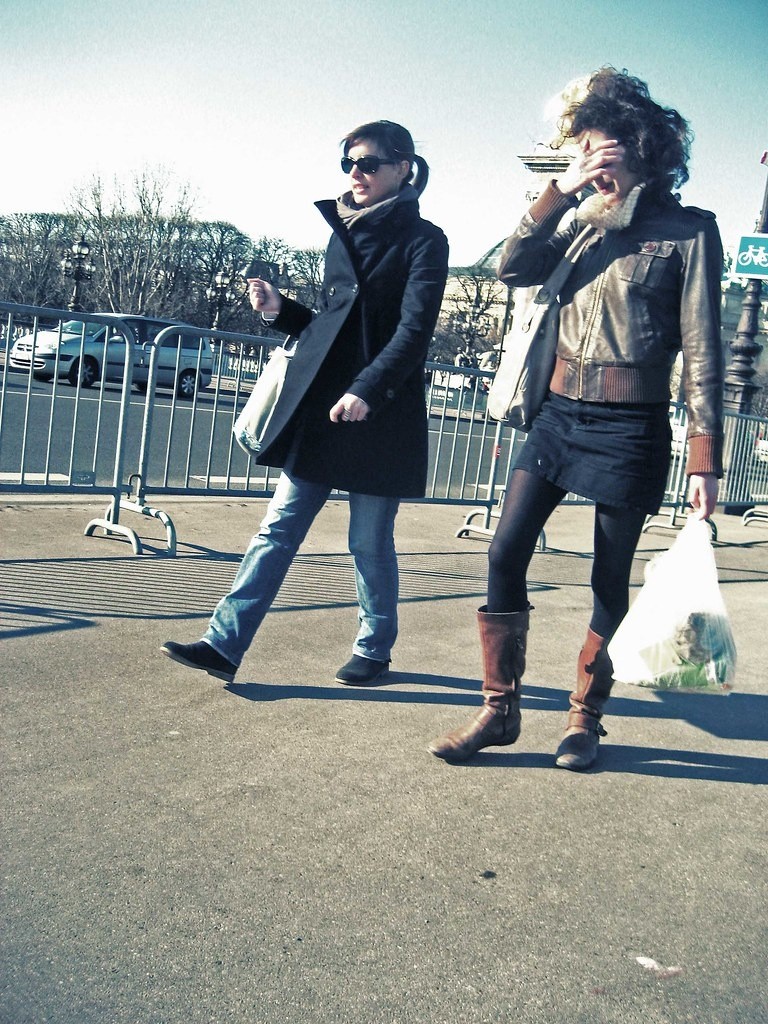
[160,641,238,683]
[335,655,389,685]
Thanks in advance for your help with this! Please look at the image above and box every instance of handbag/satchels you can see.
[607,512,737,696]
[233,309,319,457]
[486,223,595,431]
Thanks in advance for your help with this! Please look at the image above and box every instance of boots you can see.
[555,623,615,768]
[427,601,536,761]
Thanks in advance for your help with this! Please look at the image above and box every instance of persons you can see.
[159,119,449,684]
[428,67,724,768]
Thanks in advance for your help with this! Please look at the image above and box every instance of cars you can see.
[9,312,213,398]
[668,410,688,457]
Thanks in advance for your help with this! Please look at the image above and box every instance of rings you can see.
[343,410,352,420]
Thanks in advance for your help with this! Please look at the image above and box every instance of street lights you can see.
[454,295,492,369]
[205,267,236,352]
[61,235,96,312]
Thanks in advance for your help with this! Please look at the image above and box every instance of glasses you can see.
[341,154,402,174]
